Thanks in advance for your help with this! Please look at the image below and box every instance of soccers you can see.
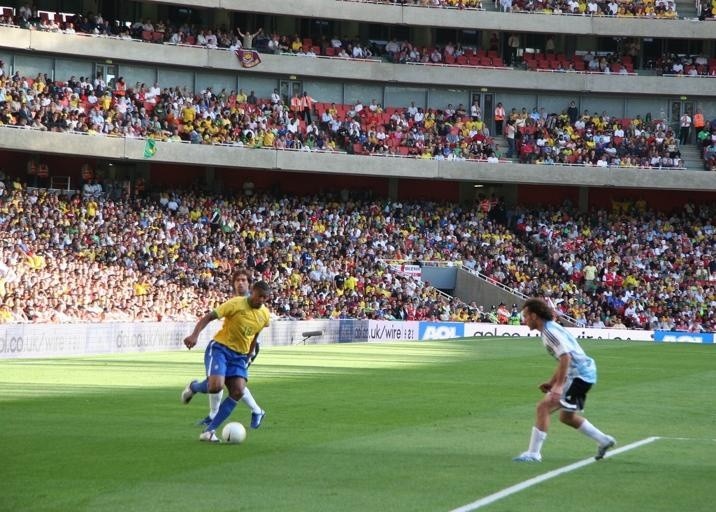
[221,422,246,445]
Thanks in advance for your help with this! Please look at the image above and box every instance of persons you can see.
[81,161,94,184]
[1,65,503,162]
[409,0,715,21]
[181,280,272,442]
[489,33,709,76]
[503,100,716,167]
[26,158,49,187]
[1,2,464,64]
[198,269,266,429]
[2,176,715,335]
[512,299,616,463]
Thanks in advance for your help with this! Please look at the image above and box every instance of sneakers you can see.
[595,436,615,459]
[196,416,211,425]
[514,453,543,463]
[181,381,198,404]
[199,430,220,442]
[251,409,266,428]
